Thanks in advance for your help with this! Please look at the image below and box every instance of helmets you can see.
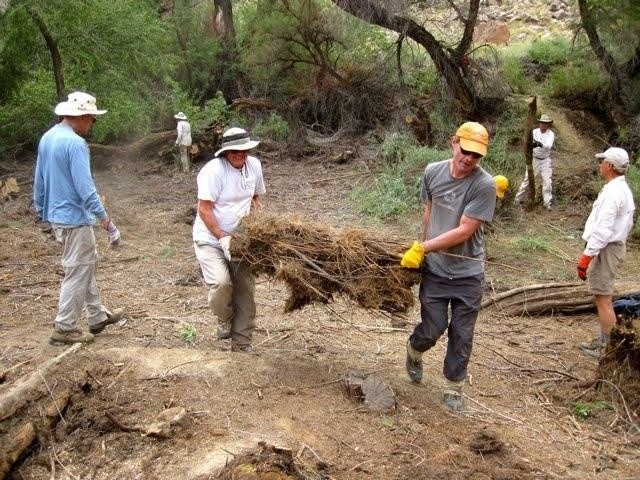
[494,175,509,198]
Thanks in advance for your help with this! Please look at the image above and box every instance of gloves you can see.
[533,139,543,148]
[400,241,425,269]
[219,235,233,261]
[577,254,593,281]
[38,224,55,243]
[101,220,121,245]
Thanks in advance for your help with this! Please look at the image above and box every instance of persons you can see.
[192,127,266,358]
[515,113,554,214]
[174,111,193,171]
[32,91,124,345]
[400,120,496,411]
[577,146,637,353]
[493,173,509,200]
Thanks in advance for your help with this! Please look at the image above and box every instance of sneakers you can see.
[405,339,423,383]
[217,319,232,338]
[580,335,605,357]
[48,329,94,345]
[89,308,124,334]
[231,344,256,352]
[441,378,465,411]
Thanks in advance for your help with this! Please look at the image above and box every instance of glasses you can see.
[92,118,96,123]
[231,150,249,154]
[460,147,483,158]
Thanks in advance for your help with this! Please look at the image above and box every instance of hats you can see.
[455,121,489,157]
[536,113,553,123]
[55,92,108,117]
[214,127,260,158]
[174,111,187,120]
[594,147,629,171]
